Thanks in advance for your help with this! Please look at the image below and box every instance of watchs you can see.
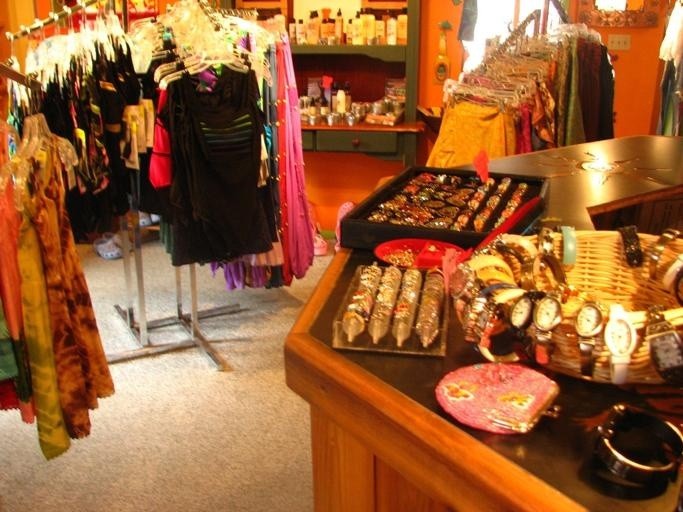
[449,226,682,387]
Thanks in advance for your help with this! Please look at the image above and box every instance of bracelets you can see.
[589,399,682,487]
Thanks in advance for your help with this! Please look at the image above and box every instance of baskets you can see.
[471,229,683,388]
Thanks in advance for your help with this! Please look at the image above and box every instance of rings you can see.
[340,258,448,347]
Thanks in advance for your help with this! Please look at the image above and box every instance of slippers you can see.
[94,235,126,261]
[138,212,151,226]
[150,213,160,223]
[104,230,134,252]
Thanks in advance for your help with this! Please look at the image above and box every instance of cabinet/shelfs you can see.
[285,38,424,178]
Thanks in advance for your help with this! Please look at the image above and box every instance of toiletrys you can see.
[287,6,407,126]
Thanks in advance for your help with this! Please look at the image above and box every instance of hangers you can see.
[0,70,81,211]
[130,1,273,87]
